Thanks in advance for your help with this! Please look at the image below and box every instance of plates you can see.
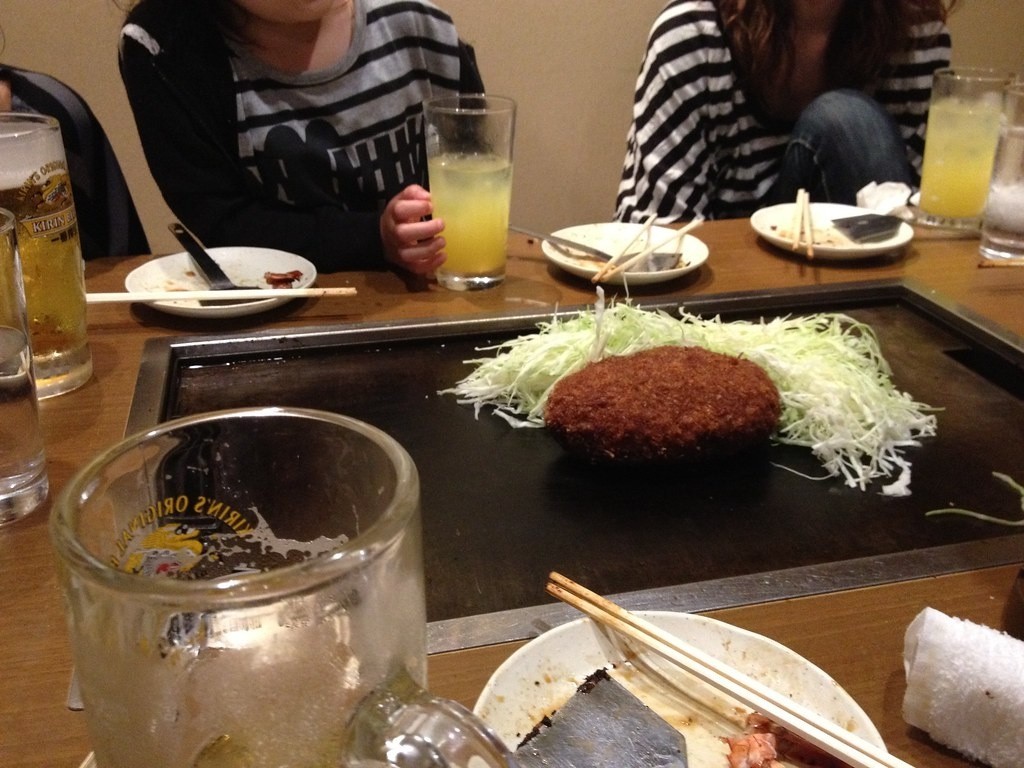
[467,610,888,768]
[750,202,914,260]
[540,222,710,286]
[123,246,317,321]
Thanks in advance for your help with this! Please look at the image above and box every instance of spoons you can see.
[508,224,679,272]
[168,222,266,302]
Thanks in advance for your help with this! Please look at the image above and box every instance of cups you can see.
[0,208,50,527]
[978,82,1024,260]
[422,93,517,291]
[48,406,516,768]
[1,110,95,400]
[919,65,1020,229]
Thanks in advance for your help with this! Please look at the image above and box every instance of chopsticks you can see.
[791,188,814,260]
[84,288,357,303]
[590,211,706,283]
[545,570,918,768]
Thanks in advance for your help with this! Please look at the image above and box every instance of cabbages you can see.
[440,270,945,496]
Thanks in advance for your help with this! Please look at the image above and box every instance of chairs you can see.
[0,63,152,260]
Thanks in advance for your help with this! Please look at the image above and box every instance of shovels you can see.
[832,213,985,244]
[168,221,271,307]
[510,666,690,767]
[507,223,682,272]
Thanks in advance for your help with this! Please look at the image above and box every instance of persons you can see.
[0,27,152,260]
[612,0,964,226]
[107,0,489,273]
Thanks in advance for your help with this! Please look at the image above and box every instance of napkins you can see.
[856,180,915,222]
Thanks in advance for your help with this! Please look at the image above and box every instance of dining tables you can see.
[0,204,1024,768]
